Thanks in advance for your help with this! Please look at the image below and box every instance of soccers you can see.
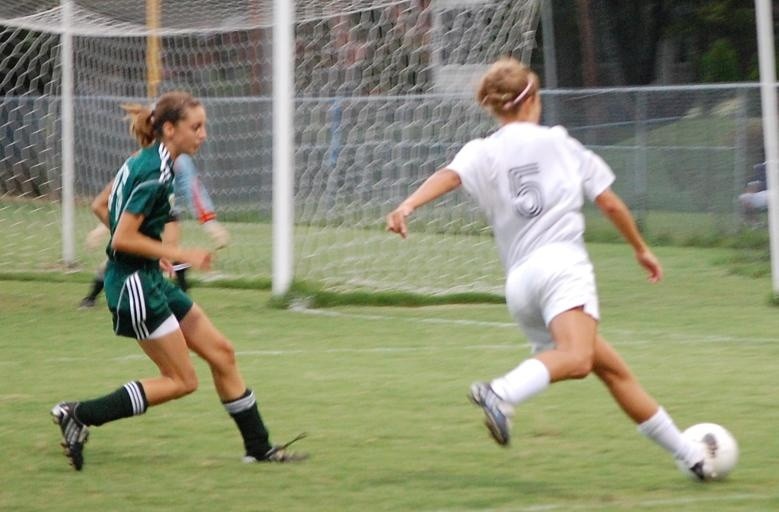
[680,423,737,481]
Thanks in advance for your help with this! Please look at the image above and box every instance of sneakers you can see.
[50,401,90,471]
[689,433,717,480]
[79,296,96,307]
[241,432,309,464]
[468,381,515,445]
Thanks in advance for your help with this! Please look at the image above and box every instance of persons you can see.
[48,92,313,473]
[74,107,230,315]
[737,150,768,222]
[385,56,717,482]
